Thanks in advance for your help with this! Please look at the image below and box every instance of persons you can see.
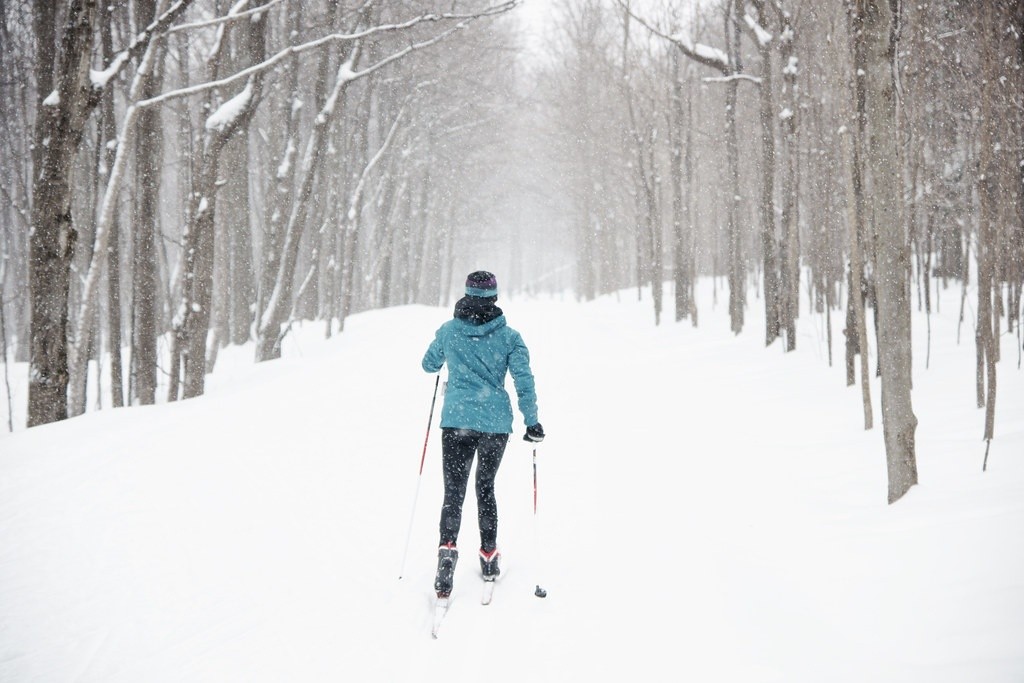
[421,270,545,600]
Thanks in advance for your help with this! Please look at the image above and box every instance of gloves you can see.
[523,423,545,442]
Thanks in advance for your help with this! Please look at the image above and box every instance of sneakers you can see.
[434,541,458,597]
[479,546,501,582]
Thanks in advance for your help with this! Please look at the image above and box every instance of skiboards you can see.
[430,581,494,640]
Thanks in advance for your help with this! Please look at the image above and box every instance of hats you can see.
[465,270,498,301]
